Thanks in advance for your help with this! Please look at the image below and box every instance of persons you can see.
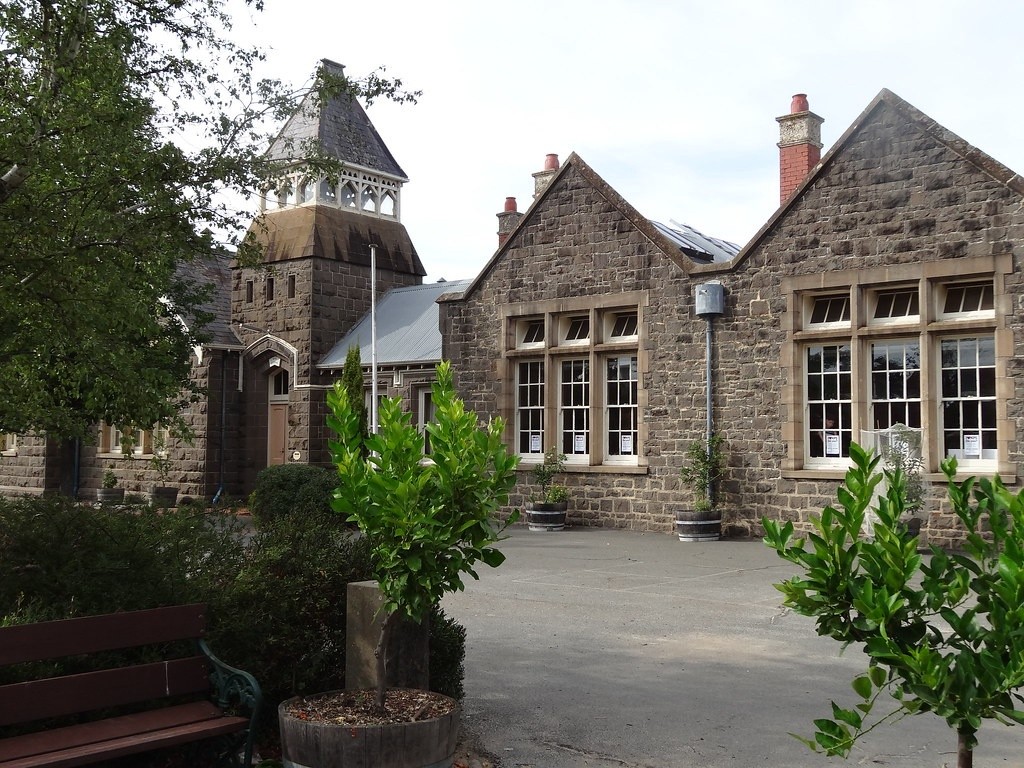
[811,414,837,458]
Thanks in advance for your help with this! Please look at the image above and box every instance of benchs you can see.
[0,604,262,768]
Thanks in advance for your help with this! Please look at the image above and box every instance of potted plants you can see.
[96,463,126,505]
[277,357,522,768]
[675,429,727,543]
[524,445,570,531]
[880,422,928,540]
[150,435,180,508]
